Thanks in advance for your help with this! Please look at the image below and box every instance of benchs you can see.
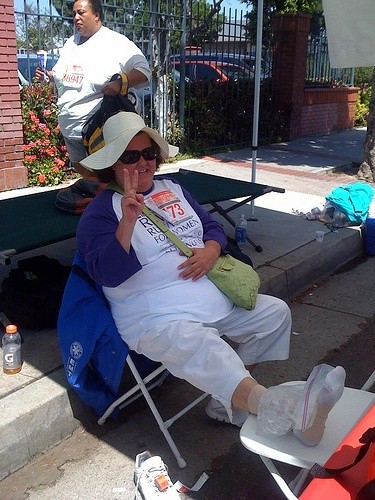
[0,169,285,344]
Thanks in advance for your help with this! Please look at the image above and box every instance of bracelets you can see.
[49,71,53,79]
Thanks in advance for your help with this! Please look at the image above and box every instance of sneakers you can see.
[133,450,181,500]
[205,398,249,427]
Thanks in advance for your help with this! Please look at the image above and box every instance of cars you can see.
[17,52,58,92]
[169,53,271,80]
[166,61,256,85]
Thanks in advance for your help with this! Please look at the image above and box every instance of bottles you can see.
[2,325,22,374]
[234,214,247,251]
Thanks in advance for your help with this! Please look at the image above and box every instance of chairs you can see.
[58,248,209,469]
[239,370,375,500]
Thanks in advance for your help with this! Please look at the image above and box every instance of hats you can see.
[78,111,179,172]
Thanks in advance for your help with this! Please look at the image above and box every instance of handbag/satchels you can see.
[206,253,260,310]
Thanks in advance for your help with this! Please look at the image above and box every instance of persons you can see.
[76,111,346,447]
[35,0,152,181]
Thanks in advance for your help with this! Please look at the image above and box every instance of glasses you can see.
[118,146,156,165]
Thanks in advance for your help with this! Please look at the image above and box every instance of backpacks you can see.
[54,178,108,215]
[80,72,140,155]
[2,255,71,332]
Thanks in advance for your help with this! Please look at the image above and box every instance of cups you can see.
[316,231,324,242]
